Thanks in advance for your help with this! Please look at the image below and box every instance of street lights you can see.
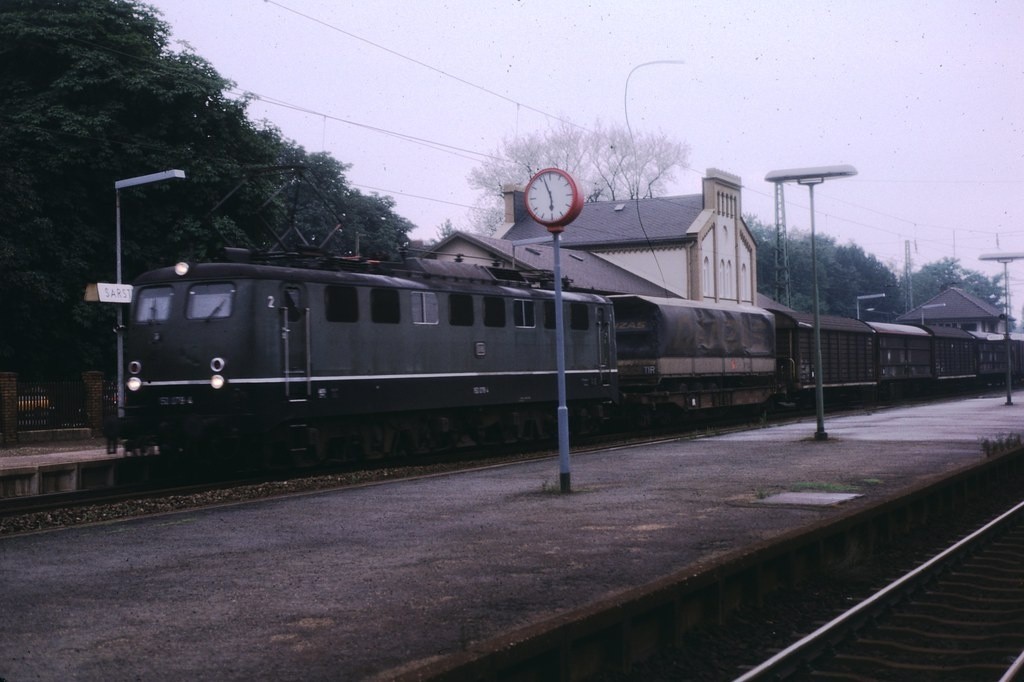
[978,252,1024,405]
[765,165,858,439]
[857,293,885,319]
[922,303,946,325]
[115,169,185,419]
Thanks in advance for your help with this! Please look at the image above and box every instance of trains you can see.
[103,247,1024,472]
